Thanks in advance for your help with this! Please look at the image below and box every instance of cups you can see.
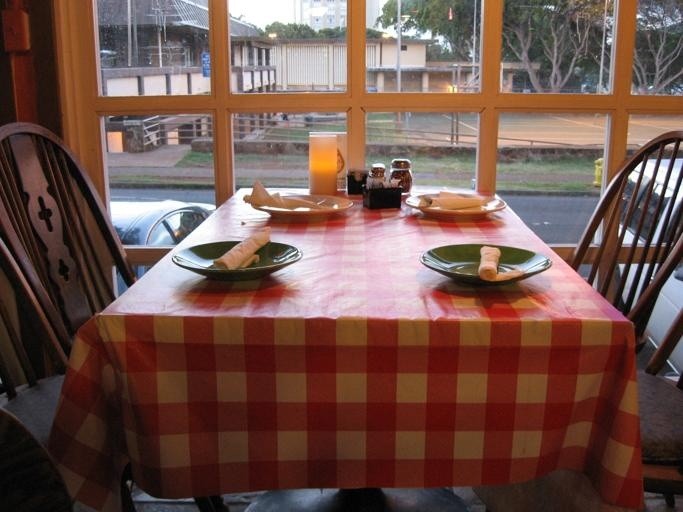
[308,134,346,196]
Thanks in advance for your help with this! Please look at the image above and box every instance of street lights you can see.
[445,64,461,145]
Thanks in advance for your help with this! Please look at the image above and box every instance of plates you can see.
[171,240,304,282]
[404,191,508,220]
[419,243,553,287]
[251,192,354,217]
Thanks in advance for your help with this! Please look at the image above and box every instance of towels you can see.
[242,180,325,210]
[419,191,485,210]
[478,246,525,282]
[213,227,271,271]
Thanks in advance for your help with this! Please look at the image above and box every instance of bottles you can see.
[388,159,413,195]
[365,163,387,190]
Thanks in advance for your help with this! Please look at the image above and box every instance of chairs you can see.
[637,307,683,508]
[568,130,683,355]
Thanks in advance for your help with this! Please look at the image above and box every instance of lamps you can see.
[308,134,338,195]
